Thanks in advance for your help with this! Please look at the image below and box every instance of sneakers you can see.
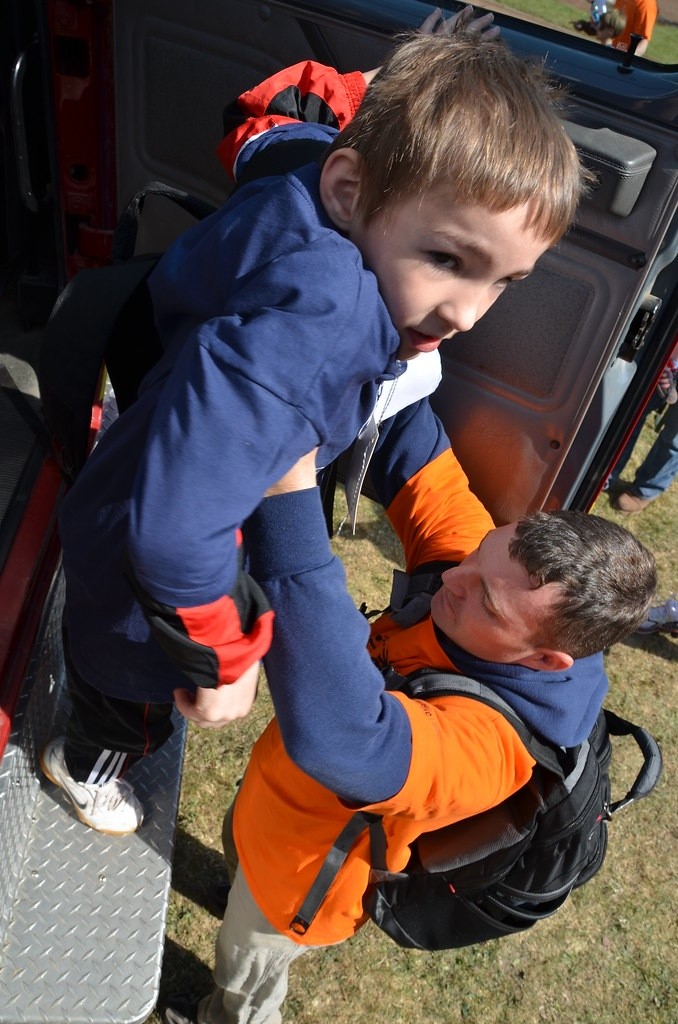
[160,998,200,1024]
[632,600,678,635]
[39,735,145,836]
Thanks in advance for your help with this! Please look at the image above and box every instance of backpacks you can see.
[366,668,663,951]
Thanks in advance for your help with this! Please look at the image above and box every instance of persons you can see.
[612,0,657,56]
[42,4,597,834]
[597,9,627,48]
[157,393,658,1023]
[602,345,678,513]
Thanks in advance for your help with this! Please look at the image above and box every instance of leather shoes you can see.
[614,481,658,515]
